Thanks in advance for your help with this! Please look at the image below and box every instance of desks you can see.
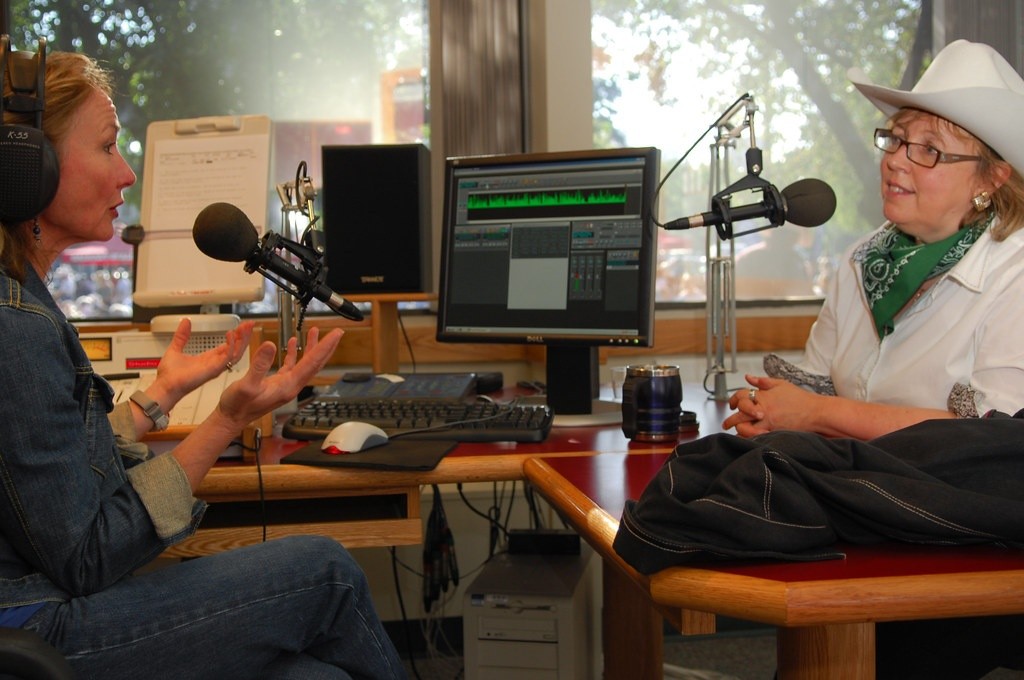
[522,452,1024,680]
[141,391,708,560]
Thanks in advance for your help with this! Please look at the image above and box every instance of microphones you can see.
[664,178,837,231]
[192,202,388,332]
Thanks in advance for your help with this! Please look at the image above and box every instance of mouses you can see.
[321,421,389,454]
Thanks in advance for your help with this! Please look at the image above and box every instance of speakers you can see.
[321,142,439,301]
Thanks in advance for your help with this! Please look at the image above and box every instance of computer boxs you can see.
[462,537,606,680]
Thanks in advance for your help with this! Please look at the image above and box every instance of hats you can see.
[848,38,1024,177]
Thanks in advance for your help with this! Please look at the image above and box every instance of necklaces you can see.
[911,288,925,306]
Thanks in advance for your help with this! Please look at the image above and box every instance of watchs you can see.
[129,390,170,432]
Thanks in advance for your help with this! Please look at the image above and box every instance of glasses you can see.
[874,127,988,169]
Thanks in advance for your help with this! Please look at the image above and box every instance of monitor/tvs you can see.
[434,147,662,425]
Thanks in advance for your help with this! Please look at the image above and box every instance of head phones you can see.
[0,33,60,223]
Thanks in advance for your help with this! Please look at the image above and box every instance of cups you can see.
[622,364,683,441]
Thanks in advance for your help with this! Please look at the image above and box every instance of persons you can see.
[0,48,412,680]
[722,38,1024,440]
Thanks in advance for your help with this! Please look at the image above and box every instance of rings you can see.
[750,389,756,401]
[226,360,233,372]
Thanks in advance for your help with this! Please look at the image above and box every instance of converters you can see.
[507,529,581,554]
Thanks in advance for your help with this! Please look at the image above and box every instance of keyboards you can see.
[282,395,554,443]
